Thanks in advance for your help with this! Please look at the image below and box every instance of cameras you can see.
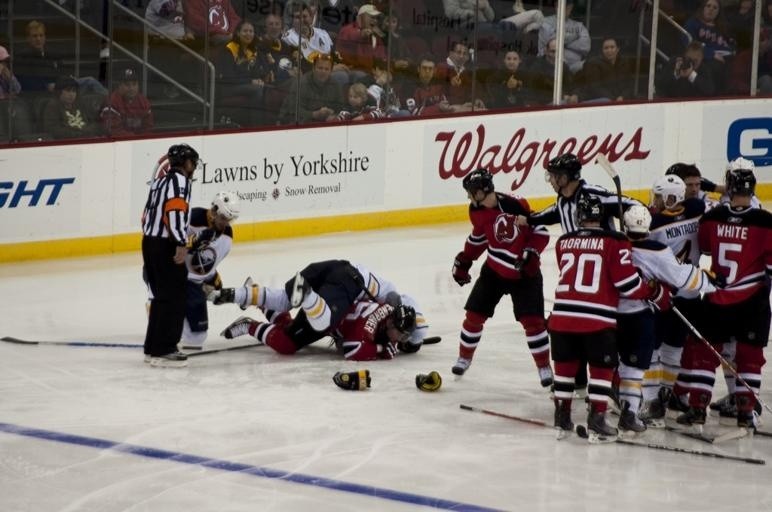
[680,56,692,70]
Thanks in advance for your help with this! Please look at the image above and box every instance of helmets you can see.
[167,145,199,169]
[463,154,755,233]
[392,303,416,343]
[212,191,241,220]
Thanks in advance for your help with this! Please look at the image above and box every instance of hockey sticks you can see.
[597,152,624,232]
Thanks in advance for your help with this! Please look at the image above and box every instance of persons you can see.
[657,0,772,96]
[145,0,634,127]
[515,153,770,464]
[142,143,204,361]
[144,191,240,346]
[202,258,441,358]
[450,169,552,388]
[0,20,153,145]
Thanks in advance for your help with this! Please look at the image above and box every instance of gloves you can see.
[452,252,472,285]
[514,250,539,277]
[416,372,441,392]
[333,370,371,390]
[397,341,420,353]
[645,280,674,312]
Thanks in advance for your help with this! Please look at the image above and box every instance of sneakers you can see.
[539,366,762,435]
[452,357,471,375]
[145,289,253,360]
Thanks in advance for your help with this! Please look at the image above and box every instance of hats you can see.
[121,65,136,81]
[358,4,384,17]
